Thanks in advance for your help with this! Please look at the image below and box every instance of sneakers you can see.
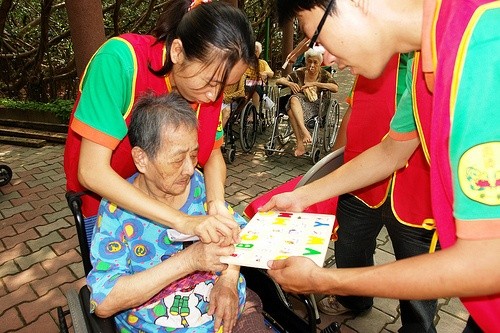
[317,295,373,315]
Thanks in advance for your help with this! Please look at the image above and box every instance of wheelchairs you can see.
[264,67,341,165]
[58,168,341,333]
[219,71,276,163]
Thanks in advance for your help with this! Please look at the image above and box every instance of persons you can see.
[258,0,500,333]
[64,0,260,333]
[298,51,336,78]
[318,50,442,332]
[245,41,274,113]
[276,48,338,156]
[86,90,274,333]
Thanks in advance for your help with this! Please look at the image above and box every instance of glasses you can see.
[306,0,333,49]
